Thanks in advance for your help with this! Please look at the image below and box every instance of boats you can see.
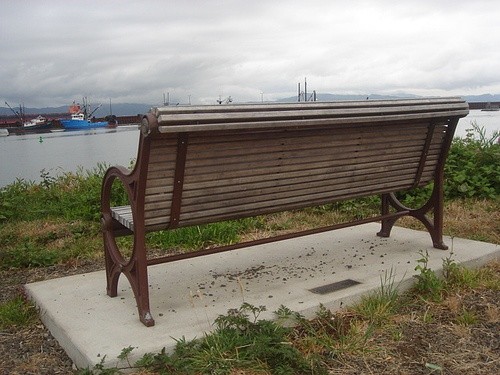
[58,114,109,129]
[4,116,54,135]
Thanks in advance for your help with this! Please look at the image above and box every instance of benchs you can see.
[99,96,470,328]
